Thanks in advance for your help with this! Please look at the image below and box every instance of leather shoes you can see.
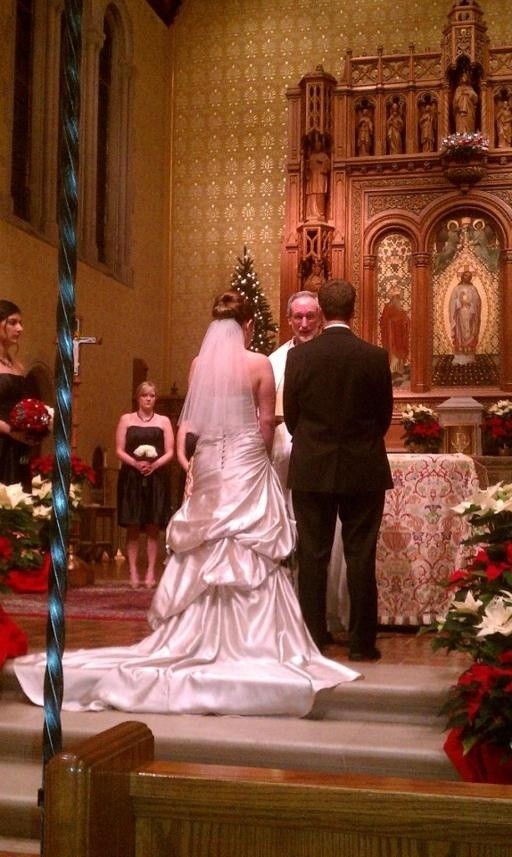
[349,646,381,661]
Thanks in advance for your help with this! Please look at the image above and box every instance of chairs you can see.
[40,721,511,856]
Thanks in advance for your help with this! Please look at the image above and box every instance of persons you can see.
[385,102,405,155]
[468,221,500,270]
[304,140,332,220]
[282,279,394,661]
[495,100,512,149]
[357,108,374,156]
[0,299,55,495]
[432,220,462,274]
[115,382,175,589]
[448,270,481,356]
[164,291,300,660]
[453,72,478,133]
[380,293,411,377]
[265,290,327,467]
[419,104,438,152]
[303,265,325,294]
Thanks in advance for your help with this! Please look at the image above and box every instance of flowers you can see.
[478,399,512,456]
[441,130,490,156]
[399,402,444,453]
[417,478,512,785]
[0,450,99,590]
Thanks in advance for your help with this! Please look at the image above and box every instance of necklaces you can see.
[136,409,155,423]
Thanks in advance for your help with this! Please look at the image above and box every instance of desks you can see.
[336,451,479,626]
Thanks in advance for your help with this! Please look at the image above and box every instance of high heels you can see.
[128,579,155,589]
